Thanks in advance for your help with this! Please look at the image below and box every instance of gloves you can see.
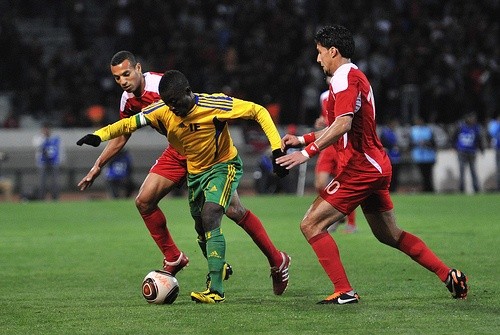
[76,134,101,147]
[272,149,289,177]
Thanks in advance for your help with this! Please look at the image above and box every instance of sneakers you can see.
[163,252,189,276]
[316,289,360,305]
[190,288,226,304]
[206,264,232,288]
[445,269,469,299]
[269,251,291,296]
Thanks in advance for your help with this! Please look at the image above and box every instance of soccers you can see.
[141,269,180,306]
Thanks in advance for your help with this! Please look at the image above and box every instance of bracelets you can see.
[305,142,320,158]
[303,132,316,145]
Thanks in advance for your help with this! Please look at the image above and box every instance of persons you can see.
[380,118,401,193]
[256,128,295,195]
[406,112,437,193]
[32,123,64,200]
[316,76,358,234]
[275,28,468,304]
[485,114,500,190]
[452,111,485,192]
[76,71,288,303]
[105,148,137,199]
[77,51,291,296]
